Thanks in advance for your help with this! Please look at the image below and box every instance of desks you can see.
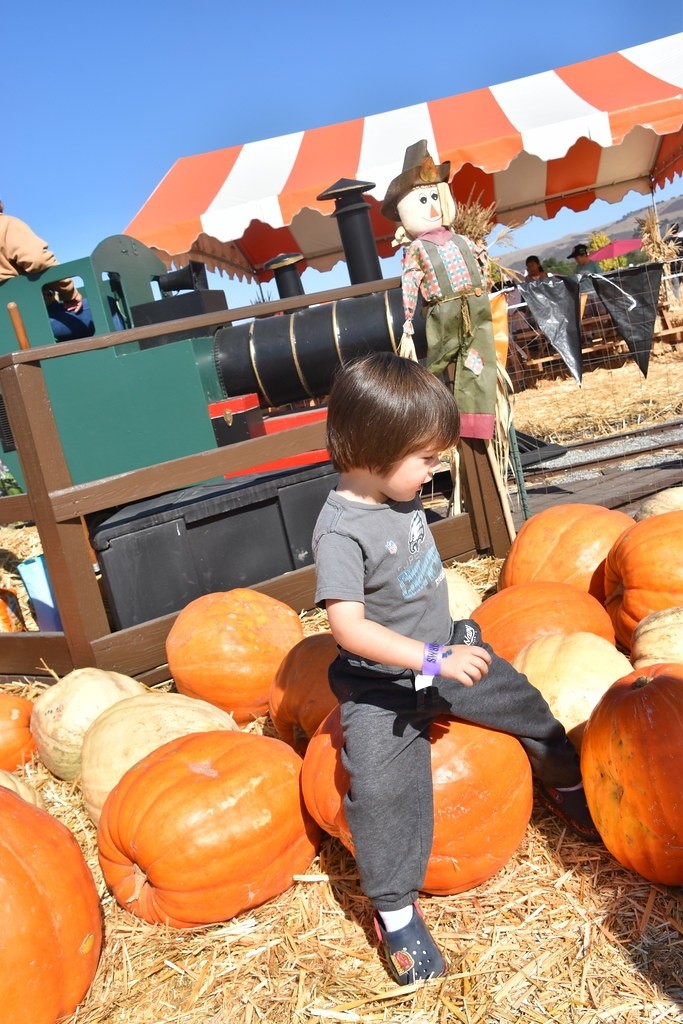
[514,300,674,385]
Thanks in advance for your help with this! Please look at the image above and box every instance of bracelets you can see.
[414,643,443,691]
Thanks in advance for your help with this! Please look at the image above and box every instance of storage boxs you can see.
[17,553,63,632]
[209,393,267,448]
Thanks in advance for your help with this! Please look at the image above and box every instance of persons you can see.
[566,243,602,277]
[489,279,533,315]
[525,255,551,282]
[1,198,131,341]
[310,355,605,986]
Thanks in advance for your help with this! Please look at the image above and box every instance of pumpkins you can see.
[0,692,46,811]
[164,587,305,726]
[80,692,244,828]
[0,784,104,1024]
[98,731,320,929]
[267,499,683,896]
[29,667,150,781]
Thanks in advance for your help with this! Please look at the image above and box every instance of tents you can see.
[122,33,683,289]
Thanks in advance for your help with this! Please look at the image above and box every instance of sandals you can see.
[372,899,448,986]
[538,782,599,839]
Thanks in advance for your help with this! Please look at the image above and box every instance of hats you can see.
[567,244,588,259]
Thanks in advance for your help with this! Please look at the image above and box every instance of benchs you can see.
[526,327,682,371]
